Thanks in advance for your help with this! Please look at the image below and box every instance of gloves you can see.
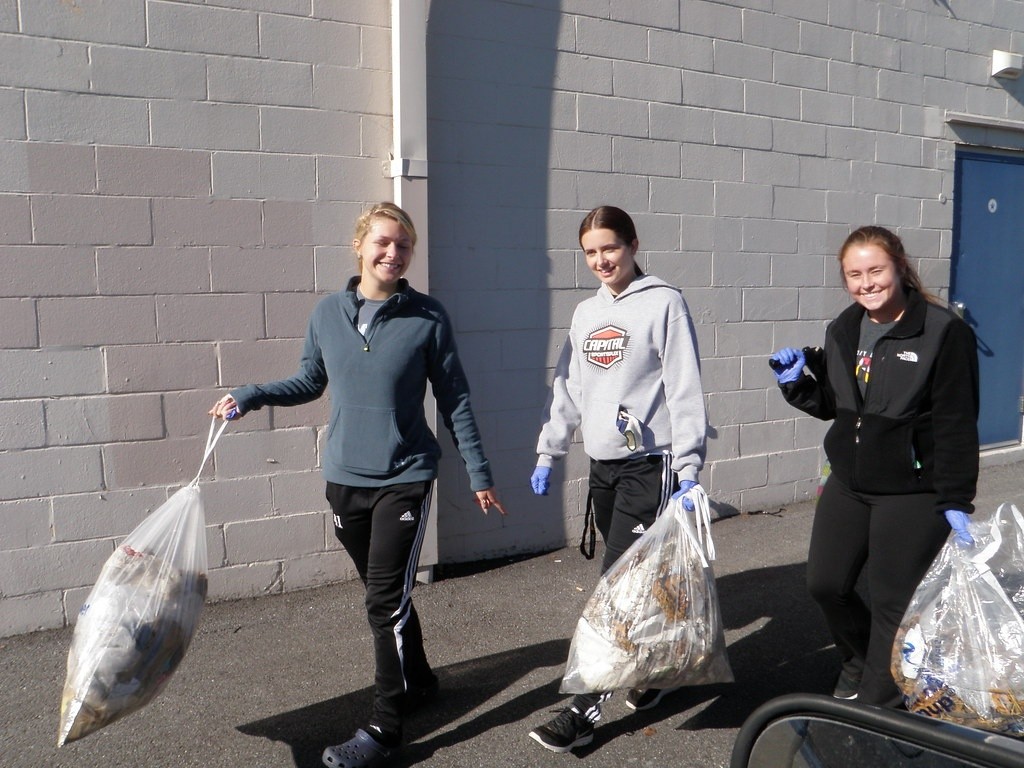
[530,468,553,495]
[769,348,806,382]
[673,480,700,511]
[944,509,974,544]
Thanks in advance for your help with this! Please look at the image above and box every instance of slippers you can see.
[322,728,400,768]
[400,675,439,711]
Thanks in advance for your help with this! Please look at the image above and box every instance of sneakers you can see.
[530,707,594,753]
[626,684,684,711]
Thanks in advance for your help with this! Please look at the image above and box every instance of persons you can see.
[769,225,980,706]
[209,202,509,768]
[529,205,708,754]
